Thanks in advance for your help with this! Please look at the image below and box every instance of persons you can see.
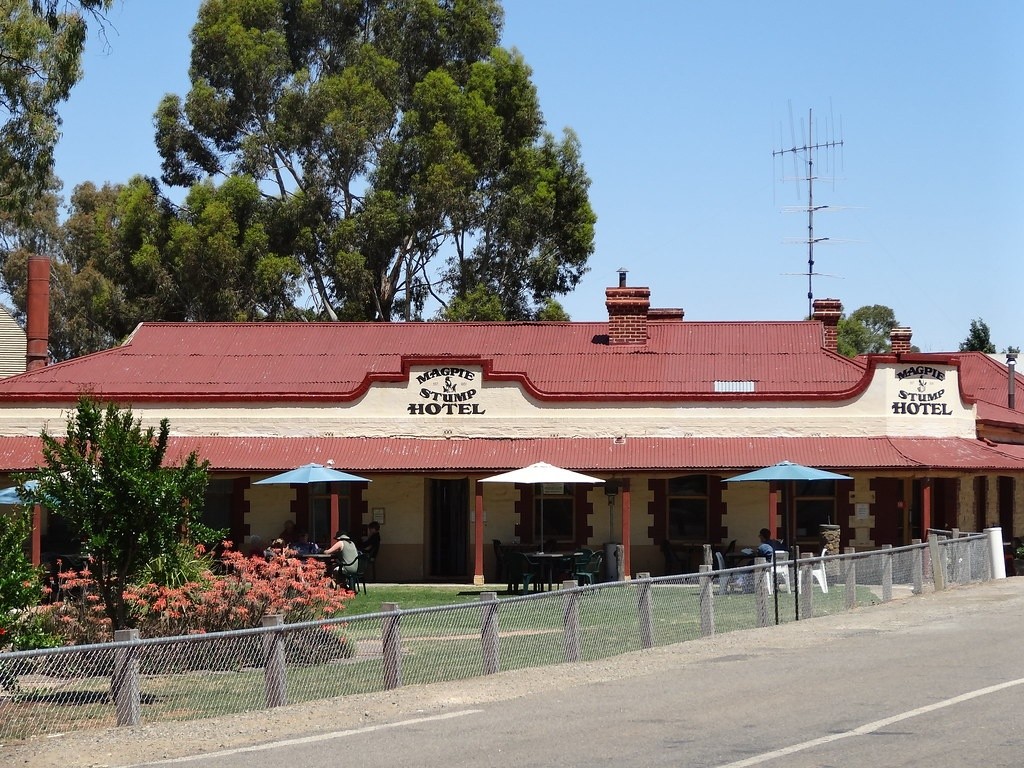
[264,520,380,595]
[729,528,783,594]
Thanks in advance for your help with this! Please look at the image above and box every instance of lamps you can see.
[602,478,619,497]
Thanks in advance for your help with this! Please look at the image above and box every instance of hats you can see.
[333,531,350,540]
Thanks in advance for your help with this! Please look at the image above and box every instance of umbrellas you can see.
[251,463,373,553]
[719,460,854,552]
[477,461,607,591]
[0,467,102,505]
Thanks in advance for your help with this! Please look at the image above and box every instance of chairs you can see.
[338,552,372,596]
[568,547,593,578]
[766,550,792,595]
[504,552,541,594]
[574,549,605,594]
[796,548,829,594]
[715,551,747,595]
[370,543,380,583]
[491,539,510,582]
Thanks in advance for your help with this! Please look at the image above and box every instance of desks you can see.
[499,543,539,552]
[533,552,566,593]
[725,547,766,575]
[300,553,334,559]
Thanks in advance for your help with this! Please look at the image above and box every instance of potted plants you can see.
[1012,547,1024,576]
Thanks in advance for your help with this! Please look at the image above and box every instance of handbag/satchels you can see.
[309,541,318,554]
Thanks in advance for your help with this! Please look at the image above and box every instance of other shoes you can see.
[340,582,350,588]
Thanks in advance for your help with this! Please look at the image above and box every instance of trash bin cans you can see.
[605,544,625,582]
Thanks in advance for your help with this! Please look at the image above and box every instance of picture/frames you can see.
[372,507,385,526]
